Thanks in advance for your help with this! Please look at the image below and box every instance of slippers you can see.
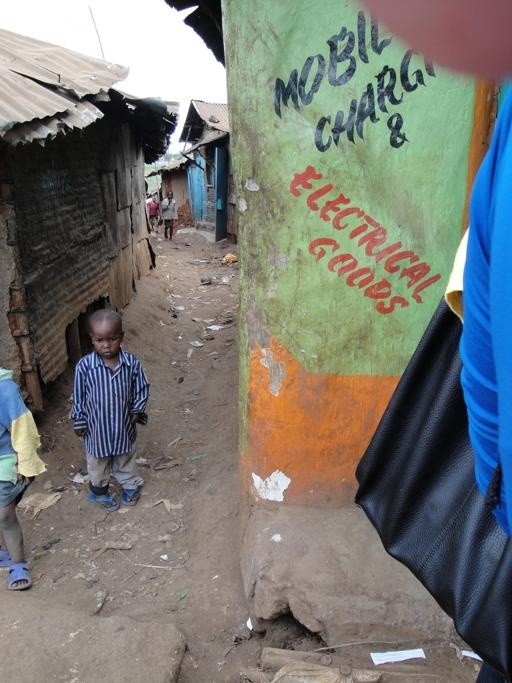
[87,489,140,512]
[0,550,32,590]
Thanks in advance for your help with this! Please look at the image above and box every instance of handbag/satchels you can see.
[158,219,163,225]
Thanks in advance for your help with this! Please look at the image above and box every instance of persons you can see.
[67,307,152,512]
[146,195,160,233]
[161,190,179,239]
[0,365,47,590]
